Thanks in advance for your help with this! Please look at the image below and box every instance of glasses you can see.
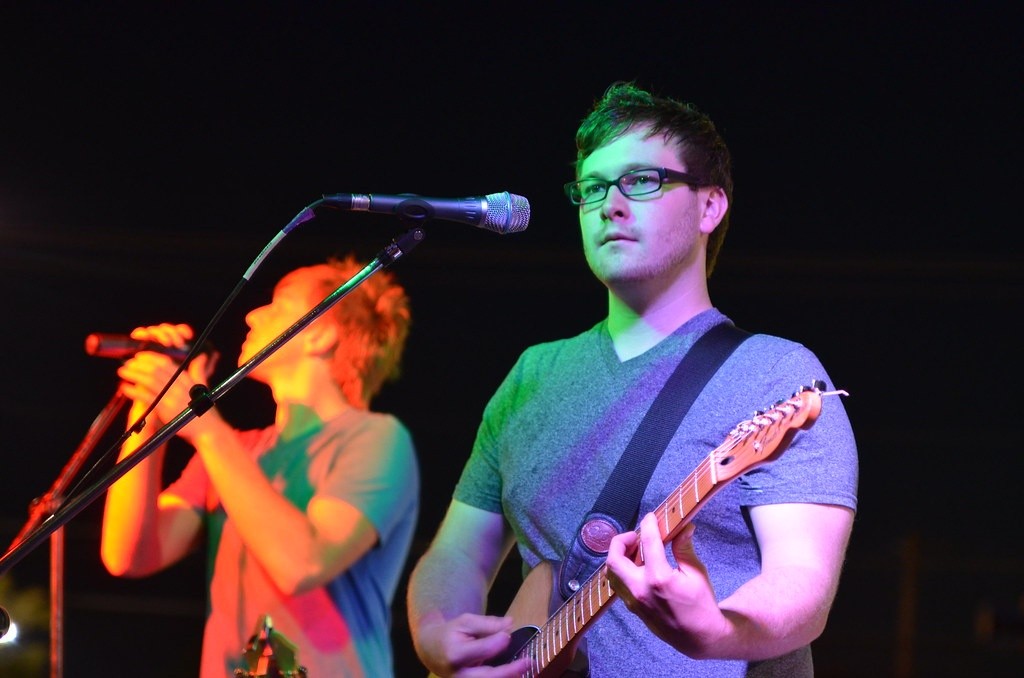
[563,167,703,205]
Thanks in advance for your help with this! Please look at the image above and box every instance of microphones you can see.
[321,190,531,234]
[86,332,215,363]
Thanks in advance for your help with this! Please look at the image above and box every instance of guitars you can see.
[429,380,828,678]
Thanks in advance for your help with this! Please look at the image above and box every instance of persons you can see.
[407,86,859,678]
[99,261,418,678]
